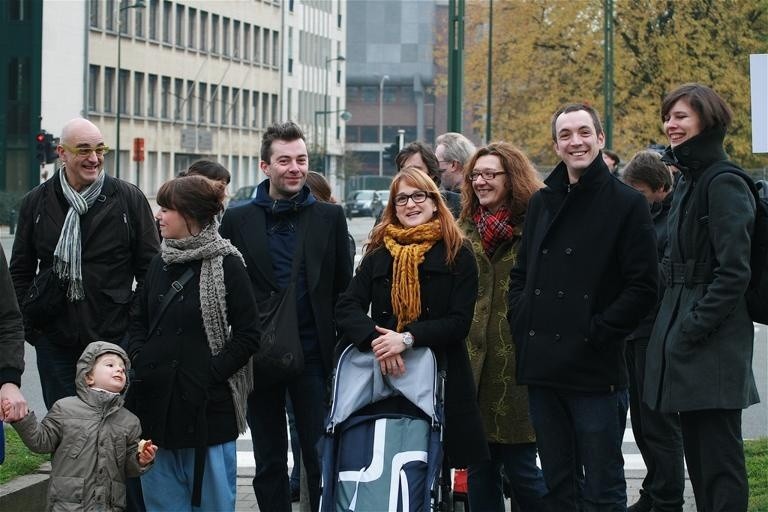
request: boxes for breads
[137,439,152,454]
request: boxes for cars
[375,188,390,208]
[226,186,259,208]
[341,188,382,220]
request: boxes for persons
[1,117,352,511]
[333,82,760,511]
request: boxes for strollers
[308,329,470,512]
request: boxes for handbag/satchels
[249,289,306,383]
[20,257,82,333]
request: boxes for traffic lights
[44,131,61,165]
[35,130,48,165]
[383,134,399,171]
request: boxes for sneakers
[627,488,686,512]
[288,477,301,504]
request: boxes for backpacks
[695,166,768,329]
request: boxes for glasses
[391,192,437,207]
[62,142,110,157]
[469,169,508,182]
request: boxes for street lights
[113,0,148,180]
[376,73,391,176]
[322,52,347,186]
[314,106,352,154]
